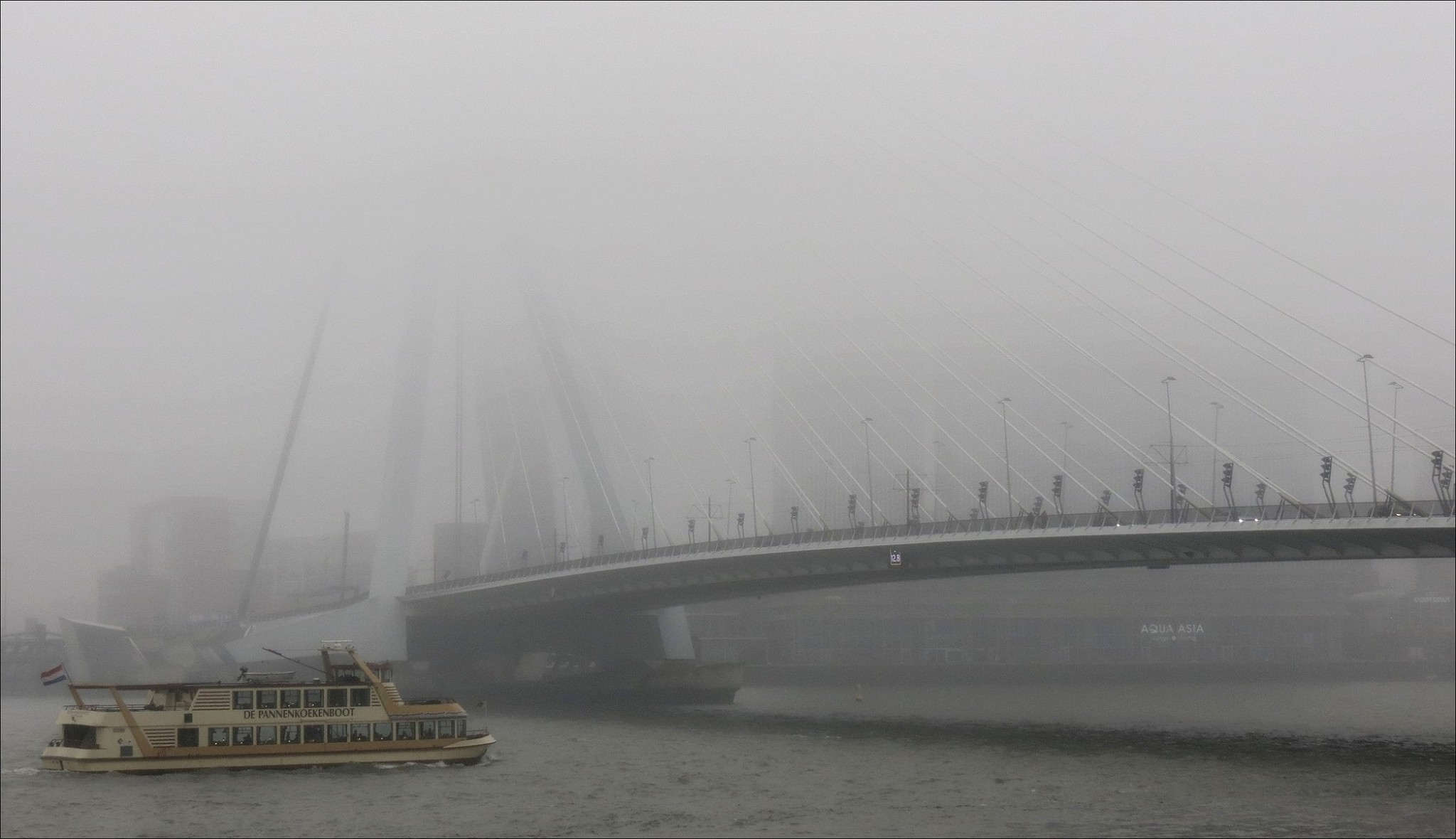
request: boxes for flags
[39,663,70,687]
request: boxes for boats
[236,665,295,684]
[37,640,493,775]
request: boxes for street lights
[861,418,876,527]
[1060,420,1073,514]
[998,396,1014,517]
[726,478,735,539]
[560,476,569,563]
[1161,376,1179,523]
[1389,381,1403,500]
[745,437,758,538]
[644,456,659,547]
[1209,400,1224,507]
[470,498,480,577]
[1358,353,1379,517]
